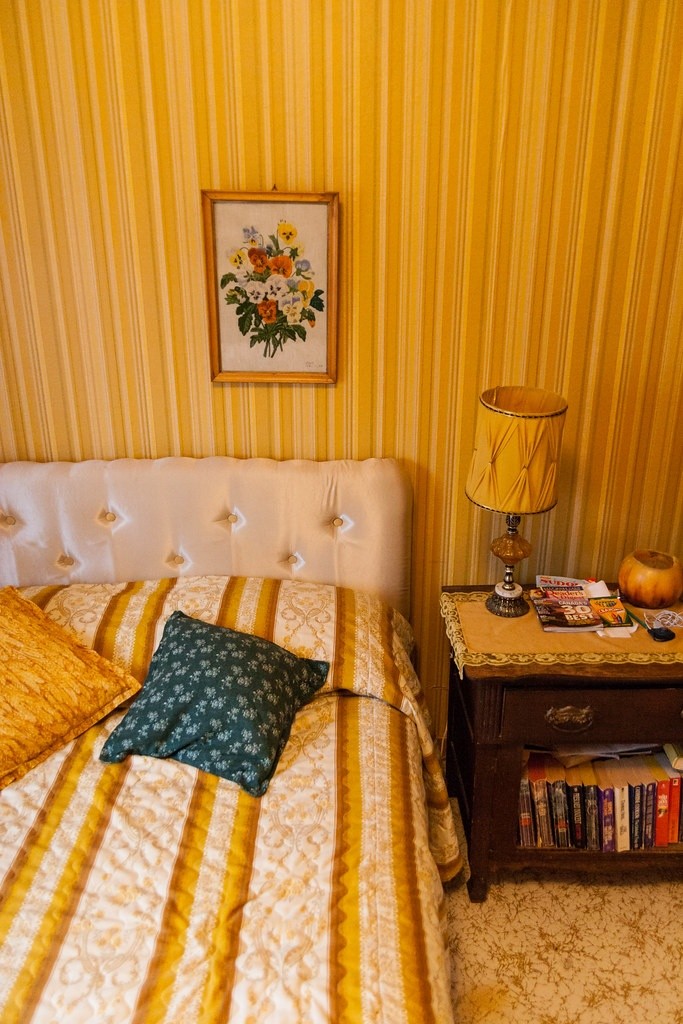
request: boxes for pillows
[0,578,139,794]
[98,610,332,797]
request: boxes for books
[529,575,634,632]
[519,752,680,853]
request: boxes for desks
[439,583,683,903]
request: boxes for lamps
[463,386,569,619]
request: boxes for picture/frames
[200,187,341,385]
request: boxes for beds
[0,455,463,1024]
